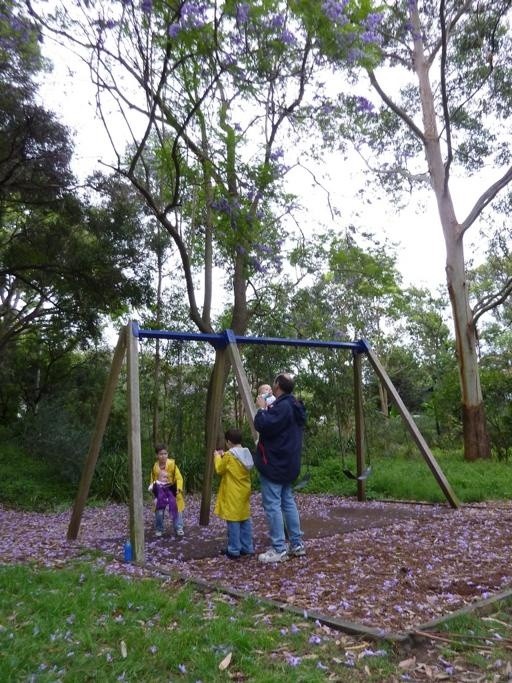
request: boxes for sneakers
[287,542,307,557]
[176,528,184,537]
[257,548,289,564]
[155,531,162,537]
[221,548,240,560]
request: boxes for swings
[140,341,182,498]
[265,344,311,490]
[331,348,373,479]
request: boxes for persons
[251,373,308,565]
[257,384,276,409]
[147,444,185,537]
[212,428,256,559]
[146,468,180,520]
